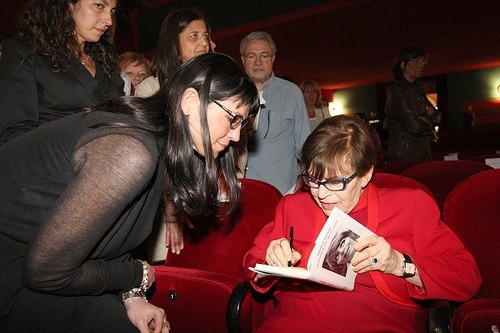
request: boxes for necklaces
[80,55,90,64]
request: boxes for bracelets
[121,287,146,300]
[139,259,150,290]
[124,298,148,307]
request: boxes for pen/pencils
[288,226,293,266]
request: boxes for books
[248,206,379,291]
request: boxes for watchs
[395,252,417,279]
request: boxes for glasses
[208,95,248,130]
[301,169,358,191]
[243,52,273,62]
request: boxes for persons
[382,47,439,161]
[325,230,359,276]
[233,32,310,195]
[0,0,481,333]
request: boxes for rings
[272,263,275,266]
[371,257,377,266]
[163,321,172,330]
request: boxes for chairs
[443,168,500,298]
[380,158,431,174]
[164,177,284,282]
[146,265,252,333]
[453,298,500,333]
[400,158,495,212]
